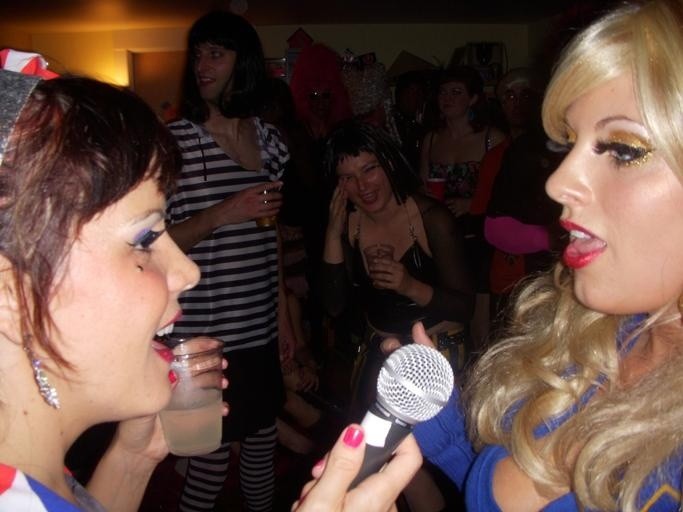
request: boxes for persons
[158,1,682,511]
[0,47,424,512]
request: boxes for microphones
[346,343,455,493]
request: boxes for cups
[256,181,280,230]
[428,177,446,203]
[164,338,226,458]
[362,244,396,266]
